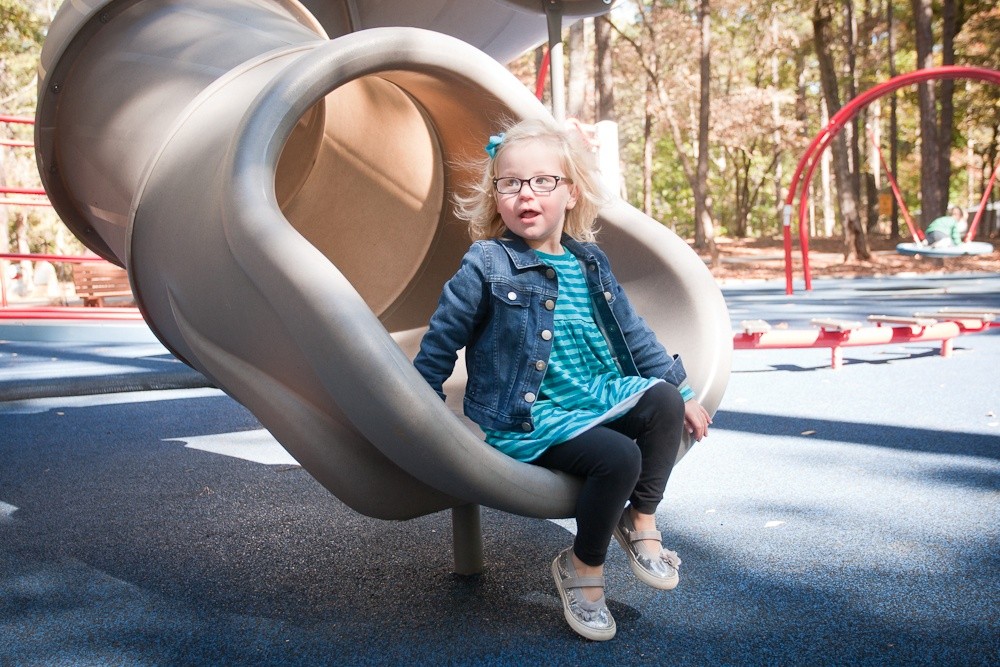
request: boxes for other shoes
[551,545,617,641]
[614,505,681,590]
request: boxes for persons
[924,206,963,270]
[412,119,713,639]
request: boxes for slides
[32,0,733,522]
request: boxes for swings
[856,104,1000,259]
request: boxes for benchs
[72,263,134,308]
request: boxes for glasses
[493,174,573,195]
